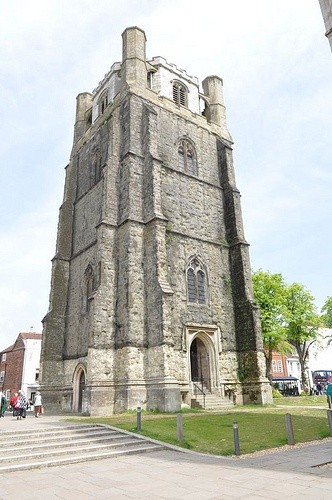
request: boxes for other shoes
[1,413,40,420]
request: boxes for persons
[33,391,42,417]
[0,389,29,420]
[278,371,332,397]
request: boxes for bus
[312,370,332,395]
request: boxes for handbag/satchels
[41,406,44,415]
[23,408,26,417]
